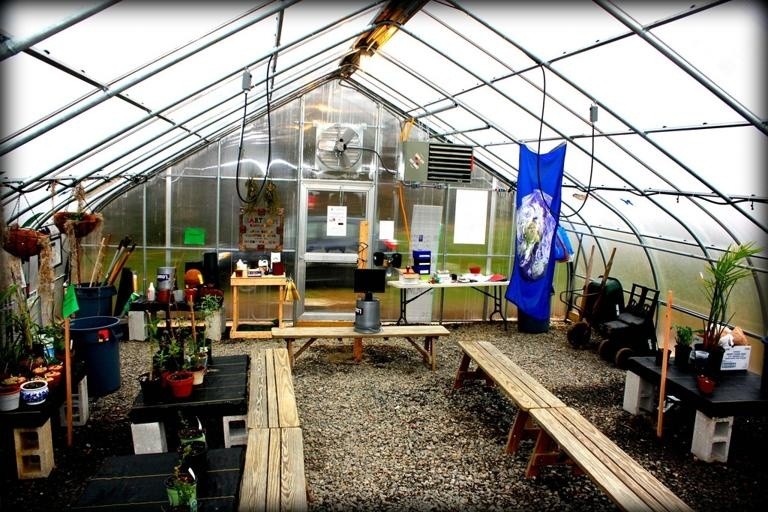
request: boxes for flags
[504,141,567,322]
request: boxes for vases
[20,380,49,405]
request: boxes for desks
[230,272,287,339]
[622,356,768,462]
[386,276,511,334]
[67,445,247,512]
[0,360,95,480]
[127,353,249,453]
[128,297,227,340]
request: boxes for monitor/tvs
[354,269,387,301]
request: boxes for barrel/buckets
[156,266,177,304]
[71,281,119,319]
[258,259,269,272]
[61,315,122,396]
[353,299,382,334]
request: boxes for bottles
[430,272,440,284]
[236,258,243,270]
[146,282,156,301]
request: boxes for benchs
[244,347,301,428]
[454,340,568,453]
[526,408,696,512]
[238,427,307,512]
[271,325,451,371]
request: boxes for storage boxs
[690,329,751,370]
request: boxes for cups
[697,374,715,394]
[241,264,248,278]
[655,347,671,366]
[272,262,283,275]
[696,350,709,367]
[236,270,243,277]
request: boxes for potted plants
[670,323,704,367]
[162,442,200,512]
[175,409,208,449]
[0,184,101,264]
[35,321,75,369]
[137,296,220,396]
[692,238,766,375]
